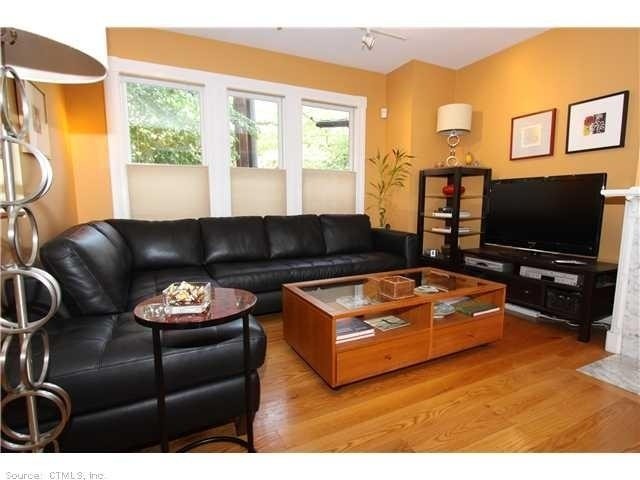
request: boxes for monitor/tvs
[484,173,607,260]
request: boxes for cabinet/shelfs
[417,167,492,270]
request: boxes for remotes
[554,258,593,265]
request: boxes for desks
[459,247,618,342]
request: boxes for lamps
[0,27,108,452]
[436,103,473,167]
[360,27,376,50]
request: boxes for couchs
[0,214,418,453]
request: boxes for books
[335,297,500,342]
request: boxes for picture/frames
[15,80,52,160]
[566,91,628,153]
[510,108,556,160]
